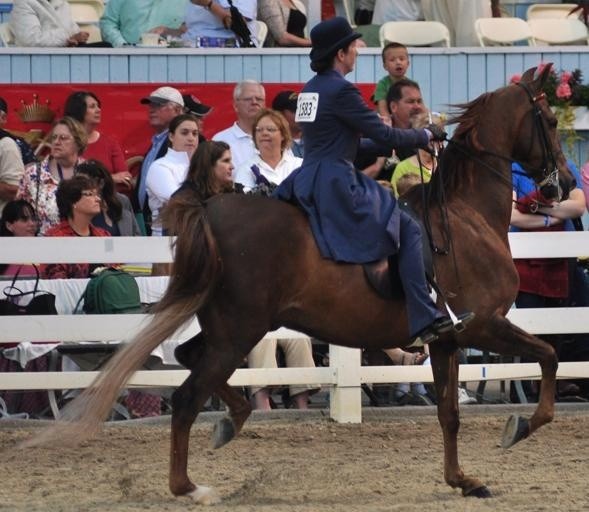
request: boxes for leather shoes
[420,311,476,345]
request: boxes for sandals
[394,351,429,365]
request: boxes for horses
[16,62,563,507]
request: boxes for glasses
[81,191,99,196]
[51,134,73,141]
[255,125,280,132]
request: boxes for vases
[549,106,589,131]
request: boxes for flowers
[509,62,588,106]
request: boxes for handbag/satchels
[0,263,59,316]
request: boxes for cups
[141,34,159,45]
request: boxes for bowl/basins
[199,38,236,49]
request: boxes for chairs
[377,3,589,48]
[0,1,113,49]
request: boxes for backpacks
[83,268,146,314]
[0,129,39,165]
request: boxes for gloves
[424,122,448,143]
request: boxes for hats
[310,17,363,62]
[140,86,185,107]
[183,90,214,117]
[271,89,299,111]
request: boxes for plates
[136,43,170,48]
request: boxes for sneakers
[458,388,477,404]
